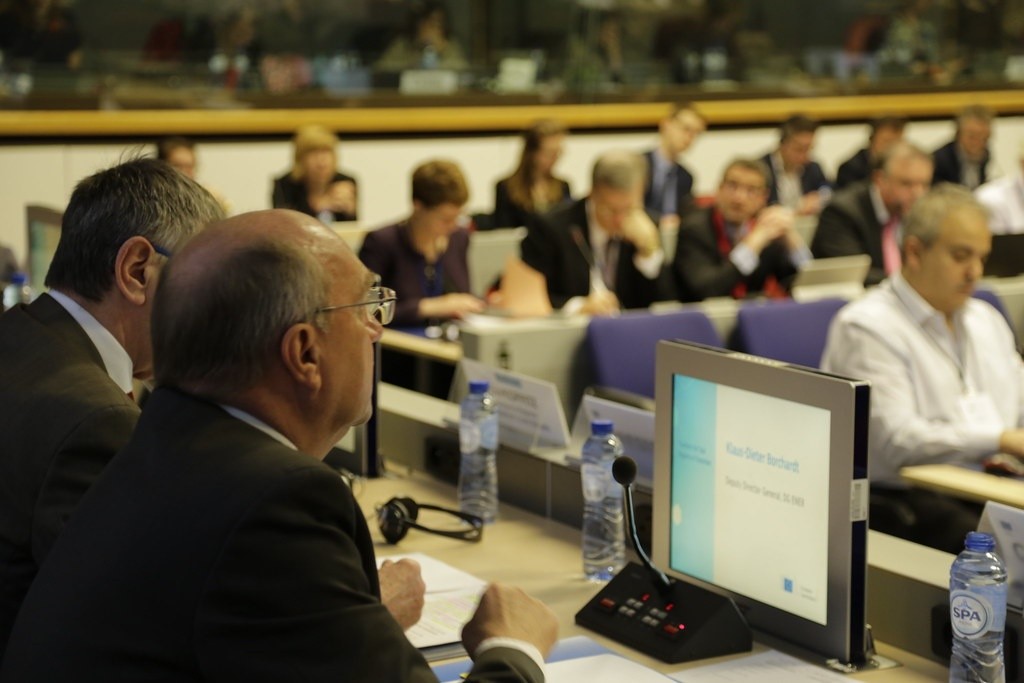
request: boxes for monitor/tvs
[324,274,383,478]
[651,338,871,659]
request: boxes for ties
[882,214,903,277]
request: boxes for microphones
[609,455,670,596]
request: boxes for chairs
[582,310,720,407]
[736,296,917,537]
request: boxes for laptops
[793,255,871,291]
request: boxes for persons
[270,124,358,221]
[156,138,196,175]
[0,157,228,657]
[932,104,993,186]
[836,116,906,188]
[542,148,667,316]
[810,141,935,286]
[673,158,815,303]
[358,160,487,331]
[758,116,828,217]
[641,105,709,229]
[493,122,572,267]
[975,157,1024,236]
[0,208,562,683]
[820,183,1024,556]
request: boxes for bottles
[579,420,625,579]
[948,532,1008,683]
[459,381,498,522]
[3,273,31,310]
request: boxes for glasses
[315,287,397,325]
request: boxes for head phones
[375,497,485,545]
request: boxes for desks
[338,210,1023,683]
